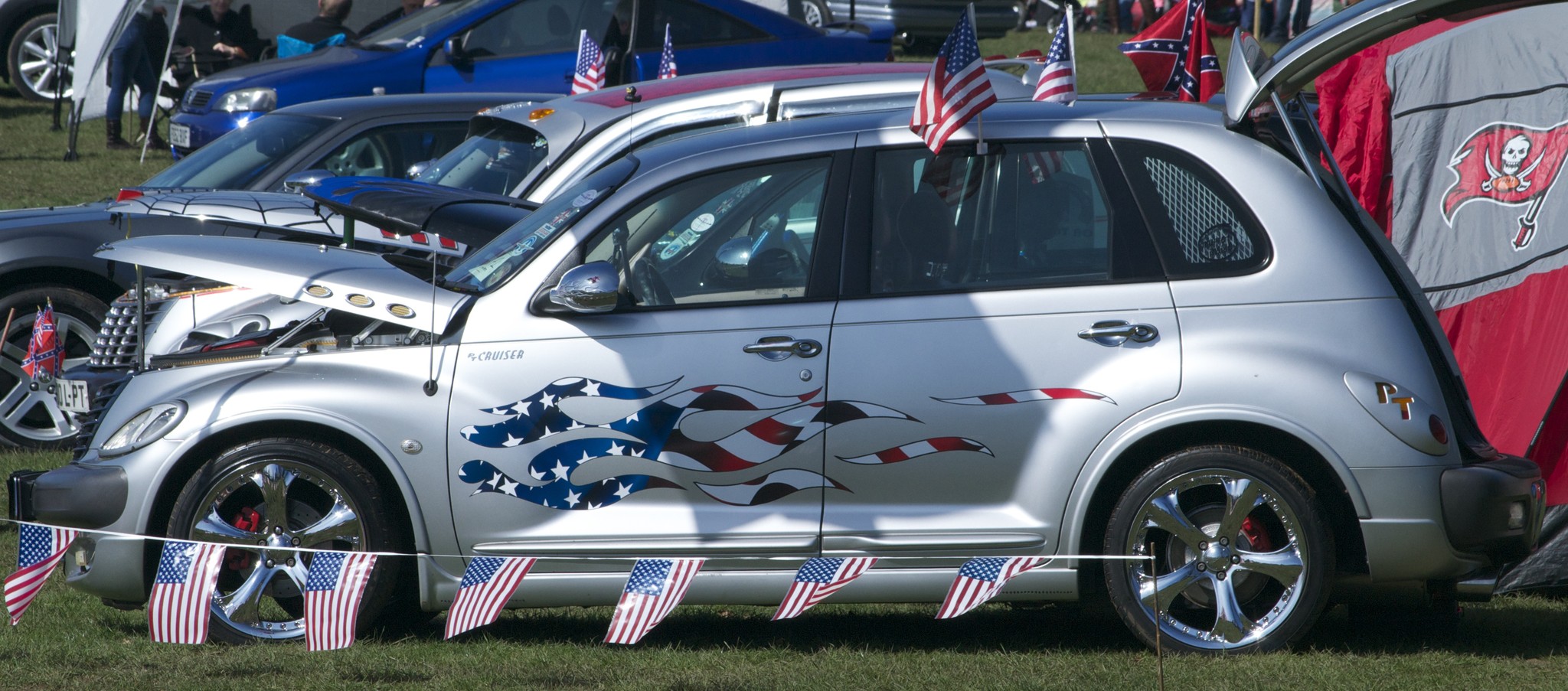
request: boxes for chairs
[123,41,203,145]
[276,31,349,60]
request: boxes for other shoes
[133,135,170,150]
[106,138,141,151]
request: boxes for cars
[0,1,1548,659]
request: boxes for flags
[305,552,377,652]
[446,557,535,639]
[20,307,64,379]
[571,33,604,94]
[602,560,703,644]
[1021,13,1075,186]
[769,558,876,621]
[3,523,80,626]
[150,542,225,645]
[924,140,1005,206]
[935,557,1039,618]
[1117,0,1223,103]
[657,29,678,79]
[909,10,997,153]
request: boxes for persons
[103,0,439,148]
[1046,0,1312,43]
